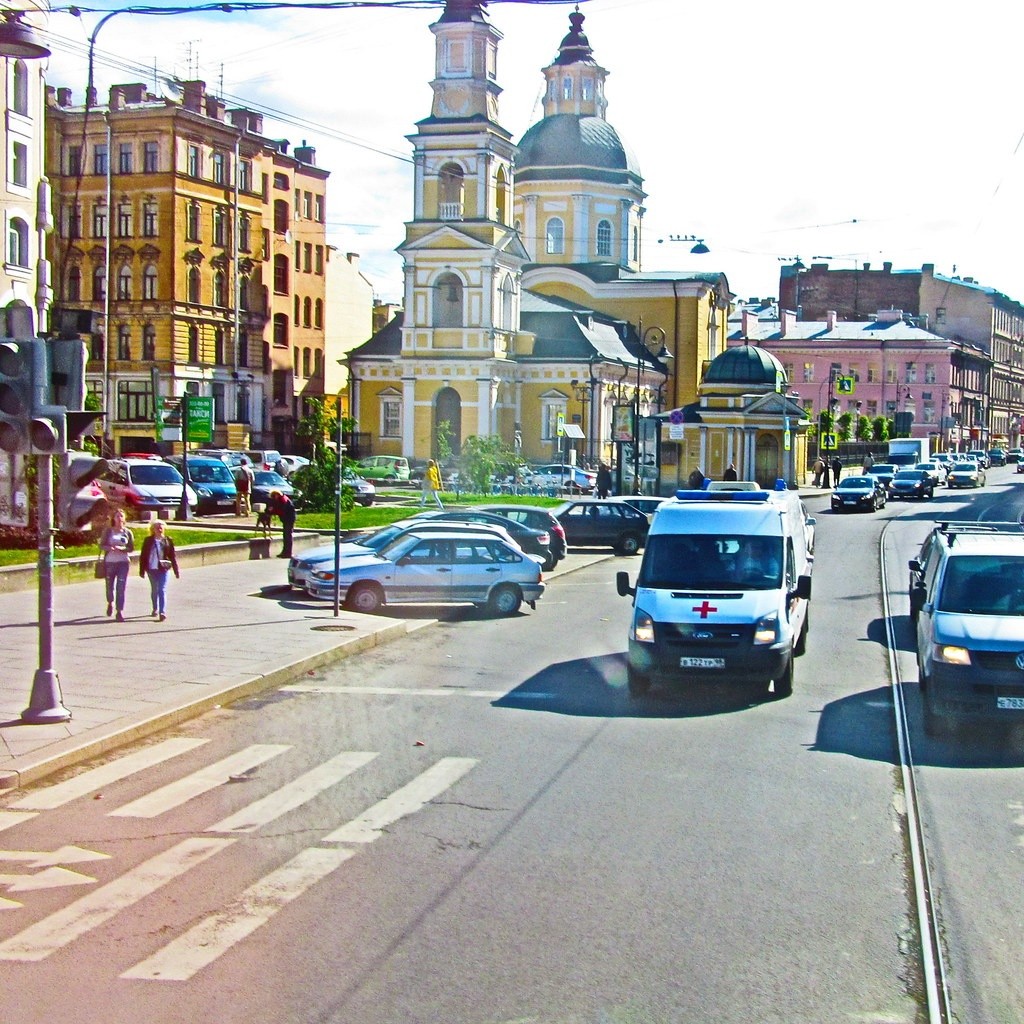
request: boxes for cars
[831,476,887,512]
[917,462,986,488]
[865,463,934,500]
[230,468,304,512]
[930,448,1024,473]
[799,499,816,552]
[281,456,598,507]
[548,497,667,556]
[289,508,553,617]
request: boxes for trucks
[887,437,930,465]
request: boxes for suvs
[909,526,1002,625]
[476,506,568,571]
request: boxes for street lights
[635,316,674,498]
[821,372,914,488]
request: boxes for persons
[268,491,296,558]
[862,452,875,475]
[419,460,444,511]
[812,457,842,488]
[139,519,180,621]
[98,508,135,621]
[725,538,779,580]
[723,463,737,481]
[689,466,704,490]
[595,464,612,499]
[234,459,255,517]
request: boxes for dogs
[255,509,273,539]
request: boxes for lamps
[793,259,805,269]
[0,10,51,59]
[691,239,709,254]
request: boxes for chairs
[436,544,457,561]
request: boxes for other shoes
[116,612,123,621]
[160,613,166,622]
[152,609,158,616]
[107,604,112,616]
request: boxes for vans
[194,448,282,472]
[917,532,1024,737]
[97,458,198,519]
[164,456,239,515]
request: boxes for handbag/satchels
[820,463,824,472]
[236,479,248,491]
[95,561,105,578]
[158,560,171,571]
[593,486,597,498]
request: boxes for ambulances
[617,477,814,696]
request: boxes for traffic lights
[0,337,68,456]
